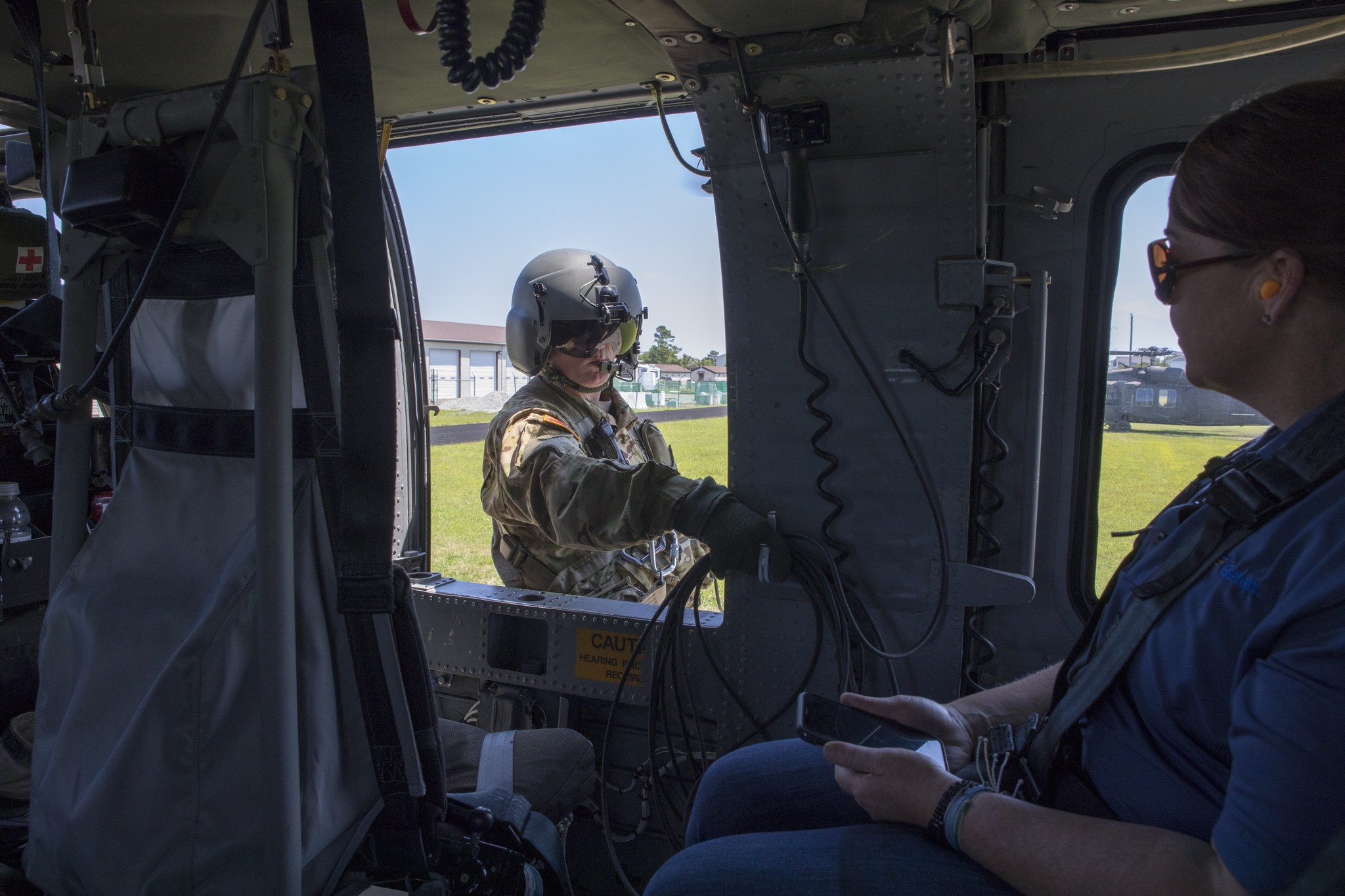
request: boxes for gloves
[699,495,789,583]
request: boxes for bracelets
[928,779,980,844]
[943,785,993,850]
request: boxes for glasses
[1148,237,1267,306]
[549,314,639,358]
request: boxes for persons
[646,79,1345,896]
[479,248,789,601]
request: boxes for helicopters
[1104,346,1272,432]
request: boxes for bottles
[0,481,32,544]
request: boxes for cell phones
[794,691,952,776]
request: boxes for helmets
[505,249,643,377]
